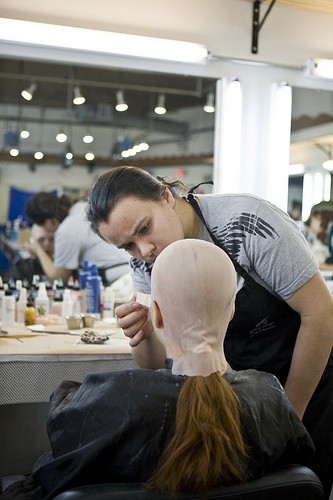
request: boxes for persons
[0,239,320,500]
[0,190,130,280]
[85,166,333,500]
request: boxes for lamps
[20,83,37,101]
[154,93,166,116]
[203,92,215,114]
[115,90,128,113]
[72,86,86,106]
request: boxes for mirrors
[286,86,333,286]
[0,55,217,316]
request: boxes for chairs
[54,465,324,500]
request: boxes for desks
[0,315,139,405]
